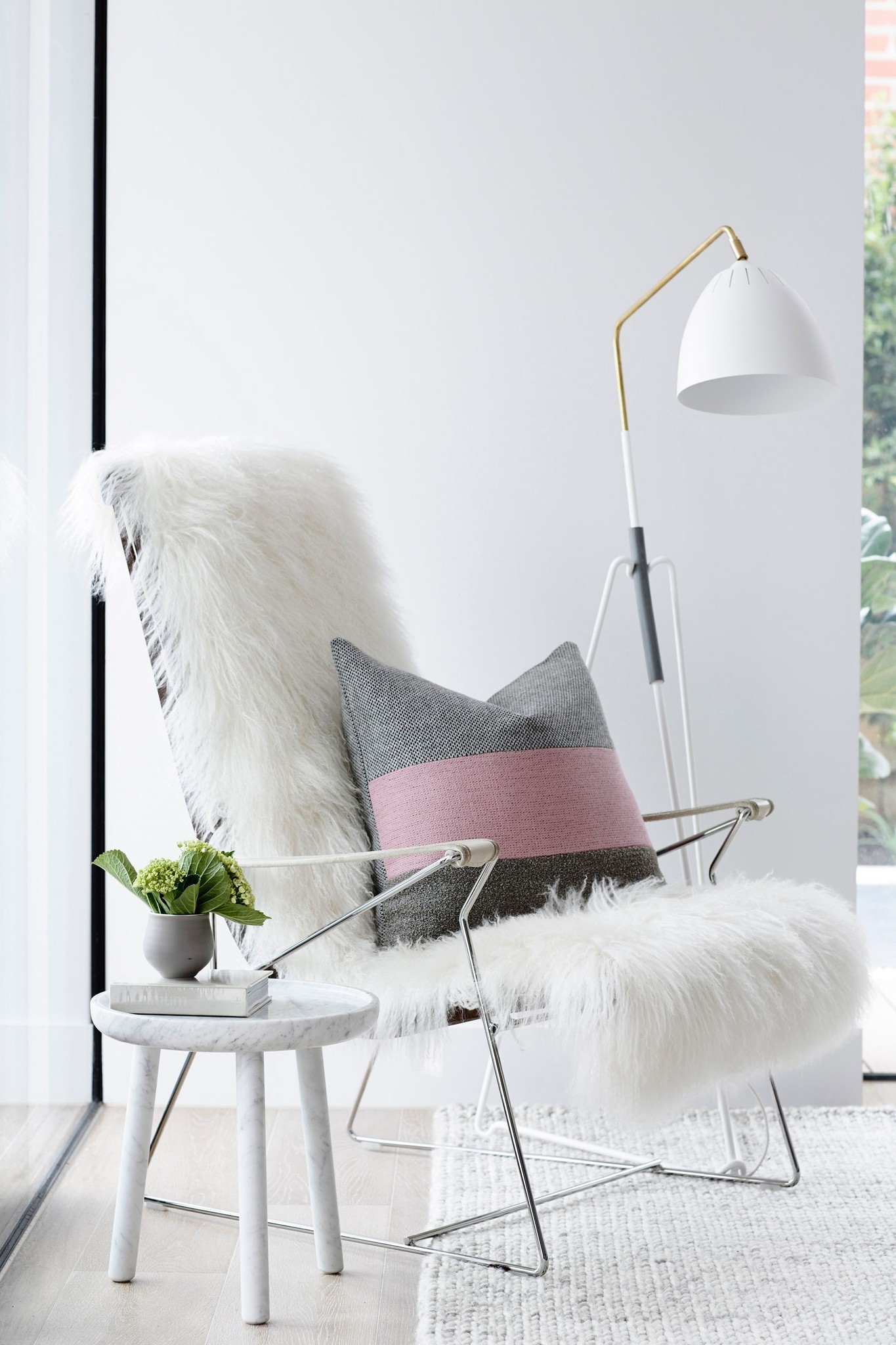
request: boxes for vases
[144,912,215,979]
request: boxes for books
[109,966,274,1017]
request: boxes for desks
[91,981,380,1326]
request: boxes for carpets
[413,1106,896,1345]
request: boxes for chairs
[69,434,870,1278]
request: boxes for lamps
[474,222,837,1185]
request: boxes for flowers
[93,840,273,926]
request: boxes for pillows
[331,638,665,952]
